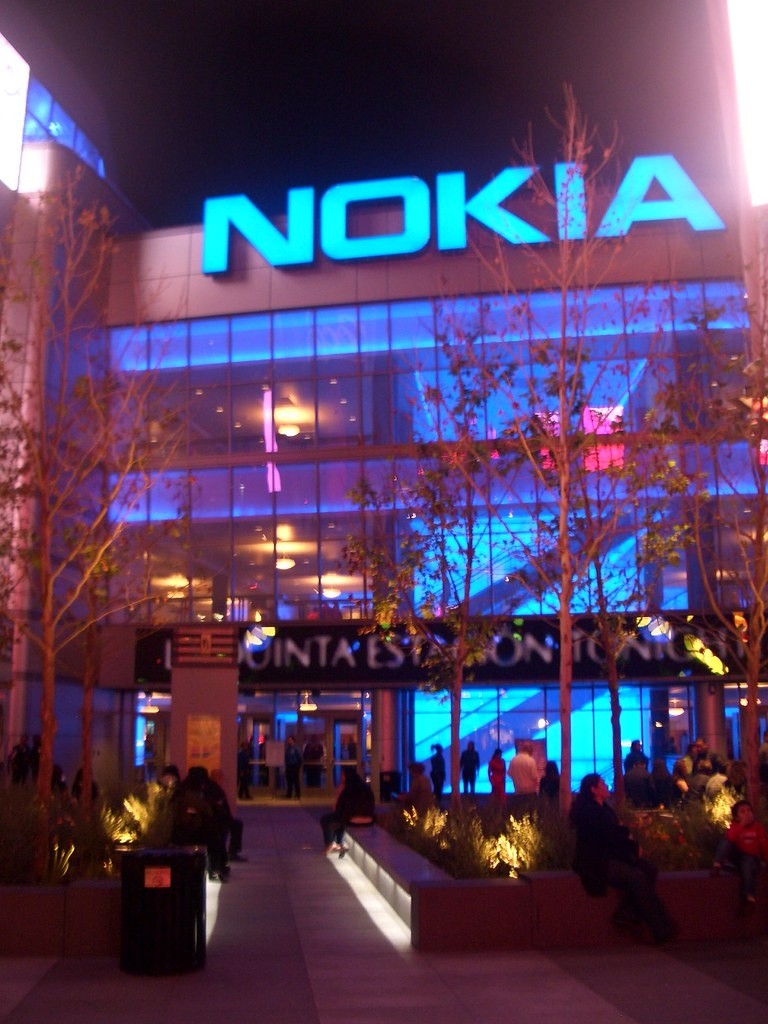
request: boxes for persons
[52,764,64,796]
[9,735,30,788]
[507,743,561,800]
[460,741,480,795]
[713,800,768,902]
[154,767,248,882]
[624,740,648,775]
[570,774,679,947]
[430,745,448,811]
[286,736,302,799]
[489,749,508,798]
[671,729,768,805]
[624,758,682,808]
[32,734,41,783]
[398,762,433,817]
[319,768,375,857]
[304,733,324,788]
[238,742,252,801]
[71,768,97,805]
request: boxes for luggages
[118,845,207,975]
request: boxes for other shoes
[229,853,246,860]
[737,898,756,915]
[330,843,349,860]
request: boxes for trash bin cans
[121,845,206,978]
